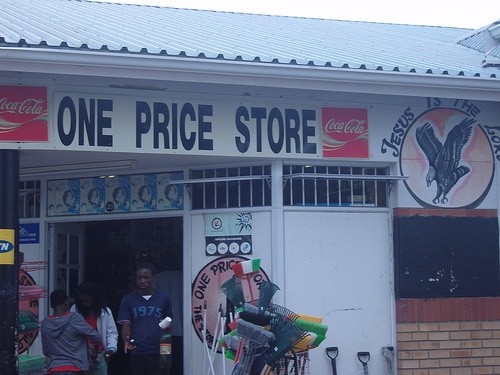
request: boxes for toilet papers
[158,317,172,329]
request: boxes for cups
[159,317,172,328]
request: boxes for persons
[40,289,102,375]
[69,260,183,375]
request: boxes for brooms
[201,258,328,375]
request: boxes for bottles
[160,332,171,368]
